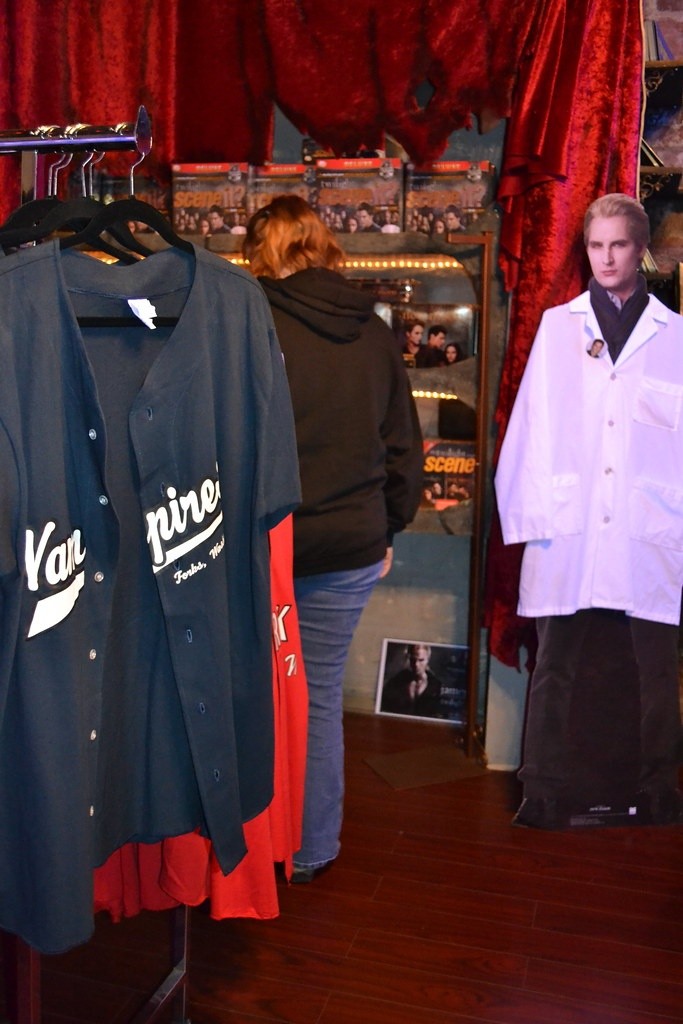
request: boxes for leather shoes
[282,858,335,883]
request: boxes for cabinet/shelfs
[0,126,135,262]
[50,123,196,264]
[1,124,154,259]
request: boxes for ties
[414,674,423,699]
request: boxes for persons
[381,643,443,718]
[586,338,604,359]
[443,342,463,365]
[241,194,426,883]
[497,192,682,828]
[414,324,448,368]
[399,318,426,368]
[127,201,480,237]
